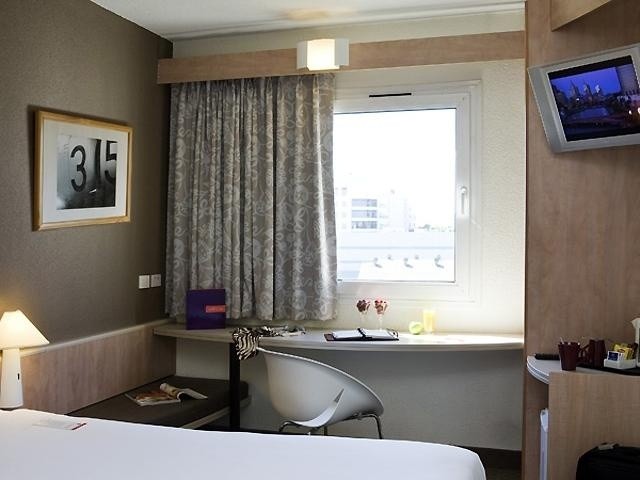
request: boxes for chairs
[254,347,384,440]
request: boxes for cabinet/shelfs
[64,375,251,430]
[526,356,640,480]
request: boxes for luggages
[575,440,638,479]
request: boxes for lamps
[0,308,51,410]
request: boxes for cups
[558,341,580,371]
[584,339,604,368]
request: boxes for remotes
[533,353,560,360]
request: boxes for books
[125,382,208,407]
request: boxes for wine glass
[374,297,388,329]
[354,296,372,329]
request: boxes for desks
[153,323,524,432]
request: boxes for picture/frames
[30,106,135,232]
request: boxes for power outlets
[152,274,163,288]
[138,274,151,289]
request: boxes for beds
[1,408,488,480]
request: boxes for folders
[185,288,227,332]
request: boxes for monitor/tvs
[526,46,636,153]
